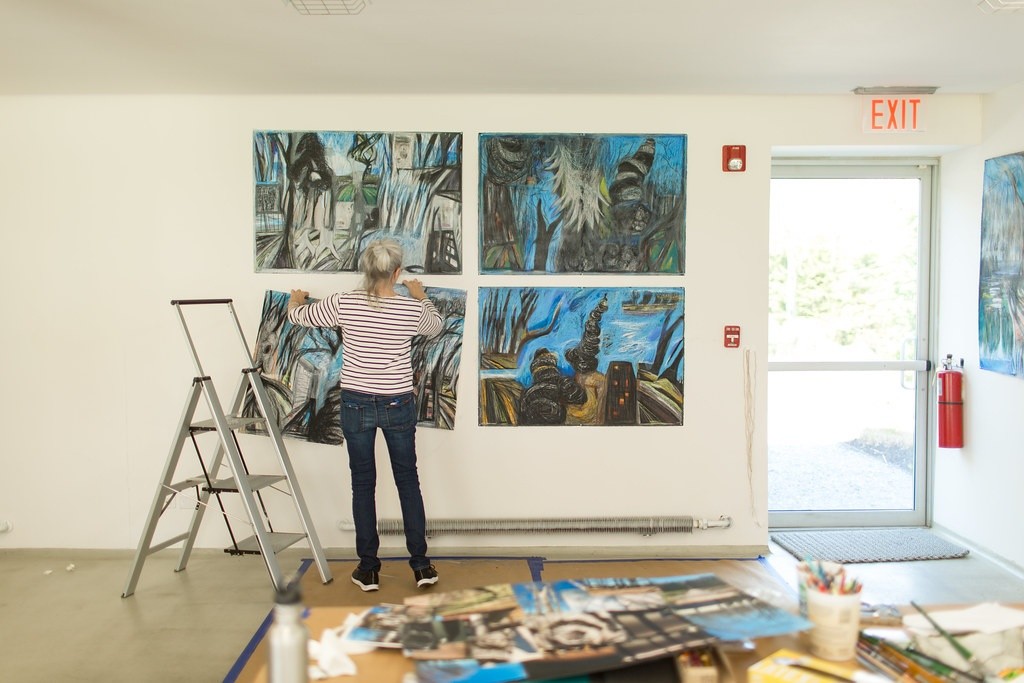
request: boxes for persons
[287,239,443,593]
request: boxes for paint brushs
[910,599,1003,683]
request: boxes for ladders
[120,299,333,597]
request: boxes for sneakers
[351,565,379,591]
[414,564,438,587]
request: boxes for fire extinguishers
[931,353,966,448]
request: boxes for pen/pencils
[798,554,865,596]
[855,627,965,683]
